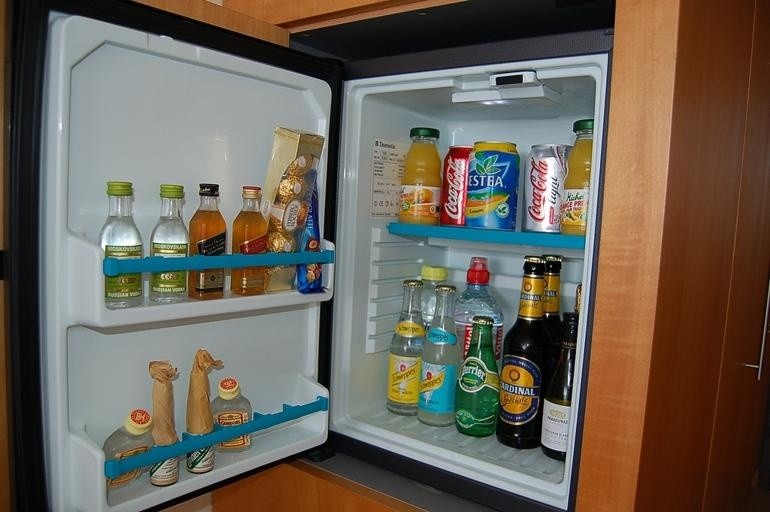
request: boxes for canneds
[441,146,472,226]
[466,141,520,230]
[524,144,573,233]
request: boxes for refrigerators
[0,0,618,512]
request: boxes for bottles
[148,184,190,306]
[453,316,500,436]
[210,377,252,453]
[231,186,267,294]
[190,183,226,299]
[103,408,155,491]
[149,360,179,486]
[399,127,443,226]
[540,312,578,460]
[417,285,464,428]
[559,118,594,237]
[496,256,548,449]
[385,279,426,415]
[419,264,448,330]
[100,181,144,309]
[541,253,565,387]
[455,257,504,377]
[185,348,224,474]
